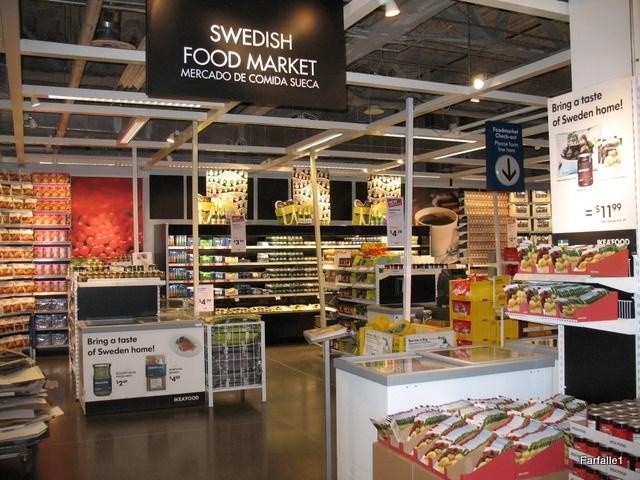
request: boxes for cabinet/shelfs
[0,172,32,366]
[67,264,168,402]
[464,191,550,278]
[152,223,431,341]
[204,319,267,408]
[33,170,72,362]
[495,239,640,480]
[329,252,465,355]
[448,278,518,345]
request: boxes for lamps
[166,132,175,144]
[469,96,481,104]
[30,119,39,129]
[382,0,401,17]
[472,76,484,90]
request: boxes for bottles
[146,355,167,392]
[628,421,640,443]
[79,273,87,282]
[135,272,143,278]
[613,419,629,440]
[149,264,156,271]
[143,272,149,278]
[154,270,159,277]
[73,260,103,272]
[587,411,602,431]
[148,271,154,277]
[110,273,117,279]
[602,410,640,421]
[587,400,640,410]
[93,273,99,279]
[598,415,614,435]
[105,272,110,279]
[88,273,92,279]
[130,272,135,278]
[160,271,165,279]
[123,272,130,278]
[577,153,594,188]
[112,255,131,263]
[137,265,144,271]
[117,272,123,279]
[99,272,105,279]
[130,265,136,272]
[124,265,130,272]
